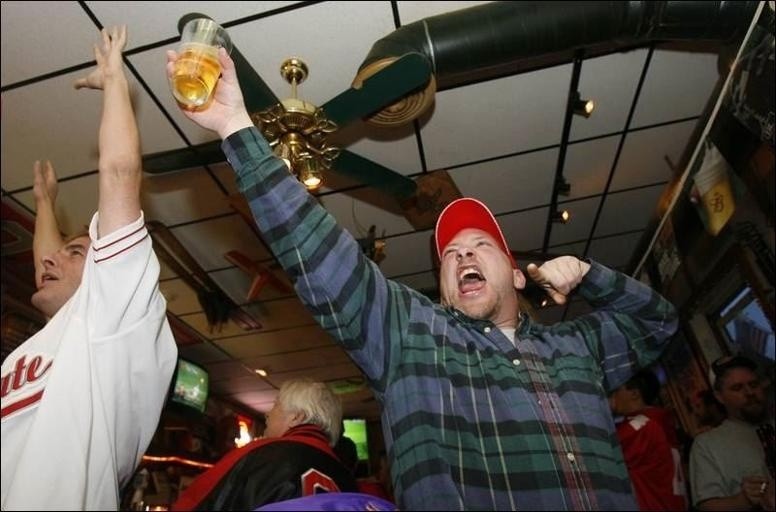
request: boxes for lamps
[264,131,327,197]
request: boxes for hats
[435,198,516,268]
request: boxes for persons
[693,390,723,427]
[166,48,680,512]
[688,354,776,512]
[171,377,362,512]
[613,368,690,512]
[1,25,180,511]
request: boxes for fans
[139,12,430,206]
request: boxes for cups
[169,18,234,108]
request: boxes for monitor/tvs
[341,417,374,470]
[171,358,210,416]
